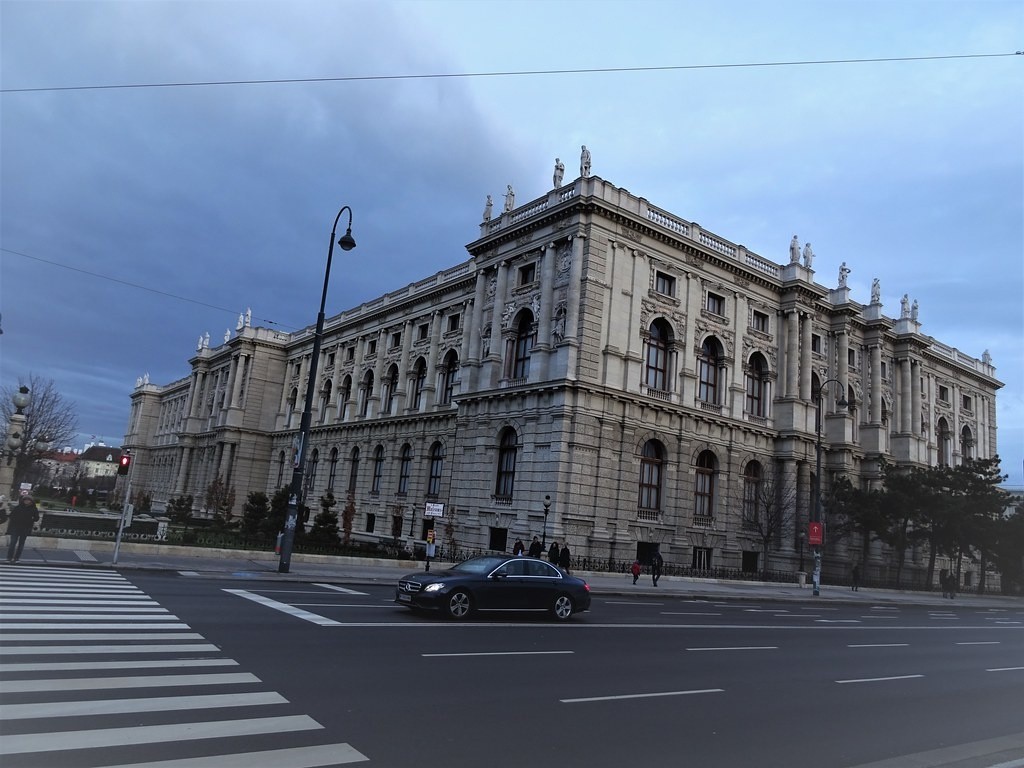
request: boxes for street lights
[408,502,417,535]
[798,528,805,572]
[814,379,849,595]
[278,206,356,574]
[541,495,552,552]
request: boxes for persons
[553,158,565,189]
[651,552,663,587]
[790,236,815,268]
[900,294,910,317]
[6,495,39,561]
[852,566,860,591]
[483,195,493,221]
[911,299,918,319]
[548,542,559,566]
[529,536,542,558]
[838,262,850,288]
[871,278,880,302]
[559,542,570,575]
[581,146,591,178]
[196,307,251,350]
[502,185,515,212]
[137,372,149,386]
[513,537,524,555]
[631,560,640,585]
[982,350,991,364]
[942,573,956,599]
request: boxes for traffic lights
[117,455,131,476]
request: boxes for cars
[395,554,592,623]
